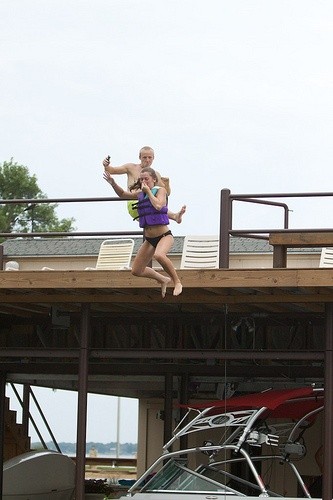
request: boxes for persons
[104,166,182,298]
[104,146,186,224]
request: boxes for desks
[269,232,333,269]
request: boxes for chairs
[180,233,219,270]
[319,248,333,268]
[85,238,135,270]
